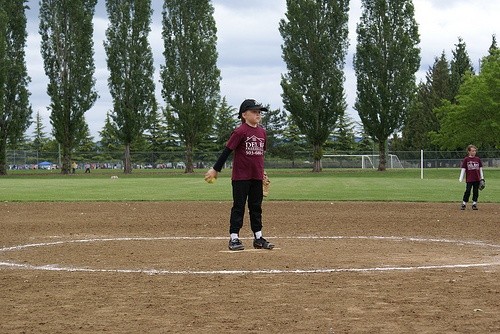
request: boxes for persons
[72,161,77,174]
[459,144,485,210]
[205,99,274,250]
[85,162,91,173]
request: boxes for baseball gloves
[478,179,485,190]
[263,171,270,197]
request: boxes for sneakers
[253,237,275,249]
[228,238,244,251]
[472,204,478,210]
[460,205,466,210]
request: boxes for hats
[238,99,268,113]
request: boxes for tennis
[209,177,216,183]
